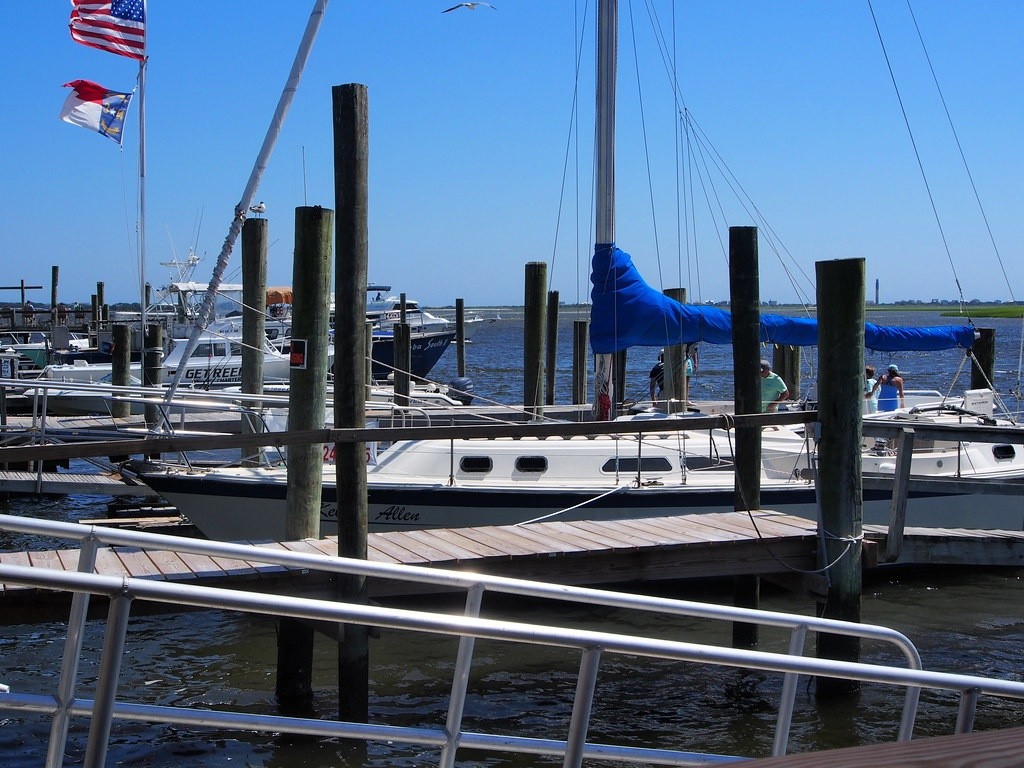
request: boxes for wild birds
[440,2,498,13]
[250,201,266,218]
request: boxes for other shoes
[686,401,697,406]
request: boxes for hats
[760,359,771,373]
[658,348,665,360]
[888,364,899,373]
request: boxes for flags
[68,0,146,61]
[61,80,132,145]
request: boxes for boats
[0,204,465,416]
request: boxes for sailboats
[118,0,1024,568]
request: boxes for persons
[864,364,905,413]
[761,360,789,413]
[649,349,664,407]
[685,342,698,405]
